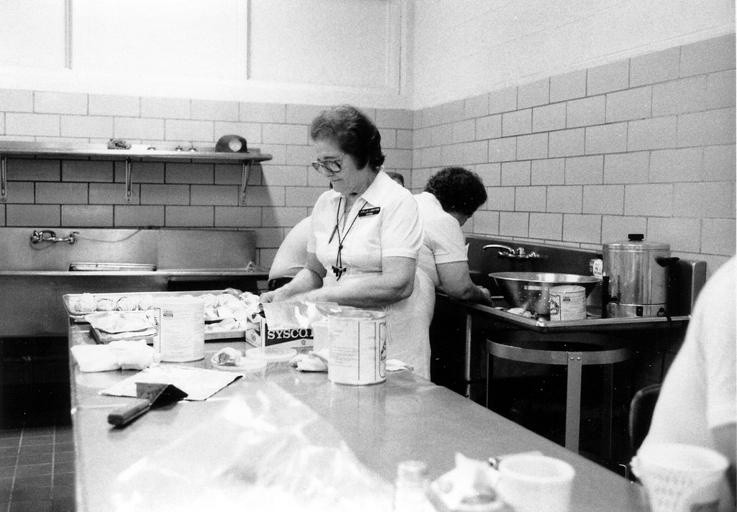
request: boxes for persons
[412,167,496,382]
[259,104,432,382]
[629,255,736,512]
[386,171,405,188]
[267,181,333,291]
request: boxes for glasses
[311,153,346,178]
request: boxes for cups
[498,455,576,512]
[637,442,730,512]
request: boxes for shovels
[108,382,188,426]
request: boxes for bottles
[393,461,433,512]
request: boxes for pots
[603,233,681,316]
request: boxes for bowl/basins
[487,272,600,316]
